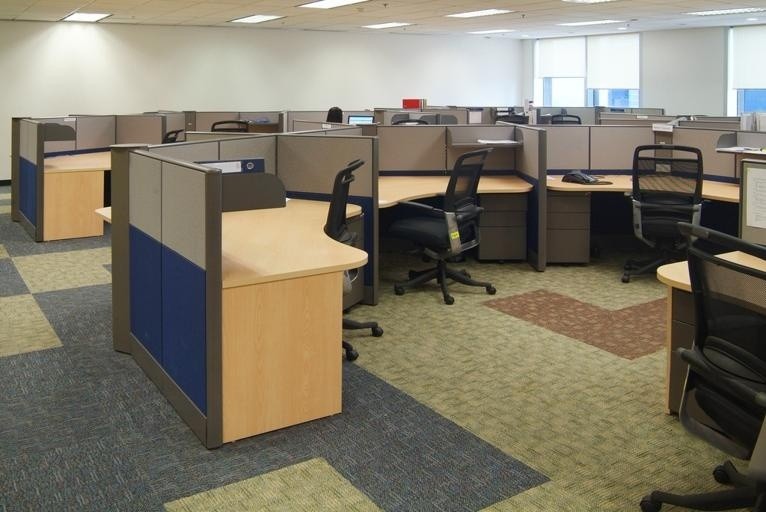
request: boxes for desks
[546,174,740,264]
[43,150,111,241]
[221,195,368,443]
[378,175,534,261]
[95,207,111,223]
[656,247,766,435]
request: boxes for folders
[193,159,264,175]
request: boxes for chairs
[325,159,383,362]
[623,145,711,282]
[495,114,581,125]
[675,222,766,461]
[392,120,428,125]
[211,120,248,133]
[162,128,185,143]
[395,149,495,304]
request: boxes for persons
[326,107,342,123]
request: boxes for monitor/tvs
[347,116,375,125]
